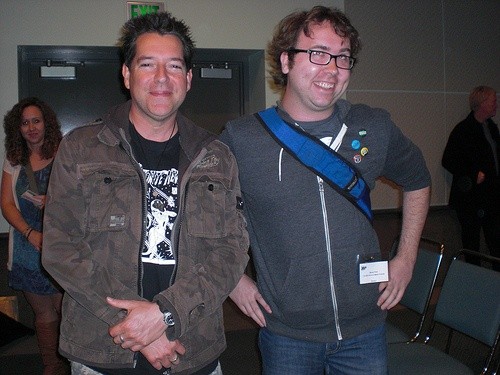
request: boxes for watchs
[156,300,175,326]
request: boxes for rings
[119,335,124,343]
[169,354,178,363]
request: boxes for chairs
[388,249,500,374]
[386,234,446,343]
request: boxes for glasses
[286,45,357,70]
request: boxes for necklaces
[127,118,177,185]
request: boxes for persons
[216,5,431,375]
[42,10,250,375]
[440,85,500,267]
[0,96,63,375]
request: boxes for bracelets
[23,225,34,241]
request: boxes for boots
[34,317,64,374]
